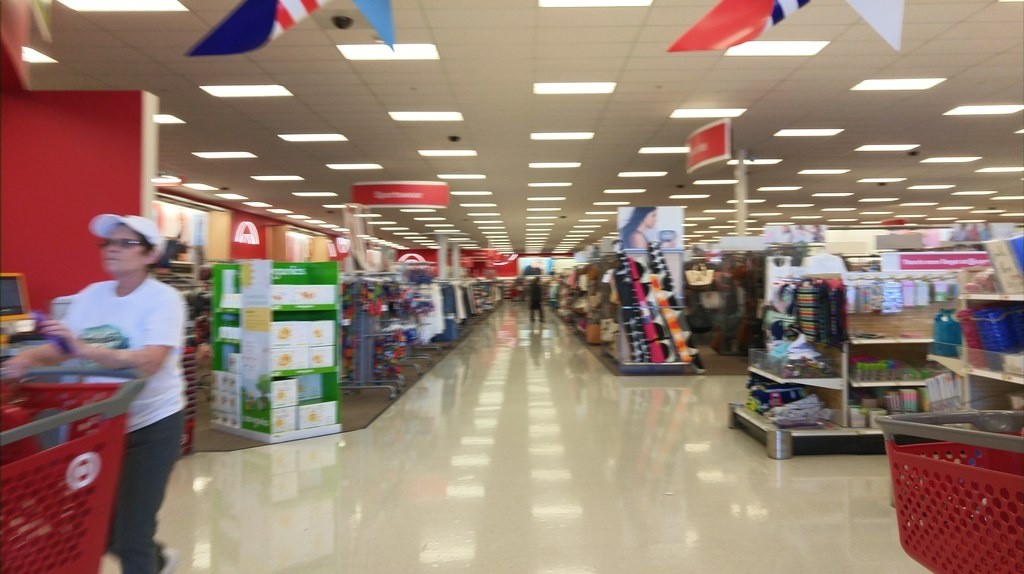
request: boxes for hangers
[382,318,419,332]
[399,278,475,290]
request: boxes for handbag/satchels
[686,305,713,334]
[699,286,723,309]
[686,262,714,285]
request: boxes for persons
[622,207,676,248]
[952,224,990,240]
[0,214,187,574]
[523,261,541,275]
[780,226,824,242]
[530,277,545,322]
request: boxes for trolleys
[0,332,146,574]
[875,410,1024,574]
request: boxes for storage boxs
[211,285,338,433]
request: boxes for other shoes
[158,550,178,574]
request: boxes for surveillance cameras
[907,152,920,157]
[677,185,685,189]
[447,136,461,142]
[219,186,229,191]
[332,16,352,29]
[878,182,888,186]
[559,216,566,218]
[328,211,334,213]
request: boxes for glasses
[96,238,150,249]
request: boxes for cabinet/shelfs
[503,205,1024,574]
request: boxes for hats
[88,214,164,246]
